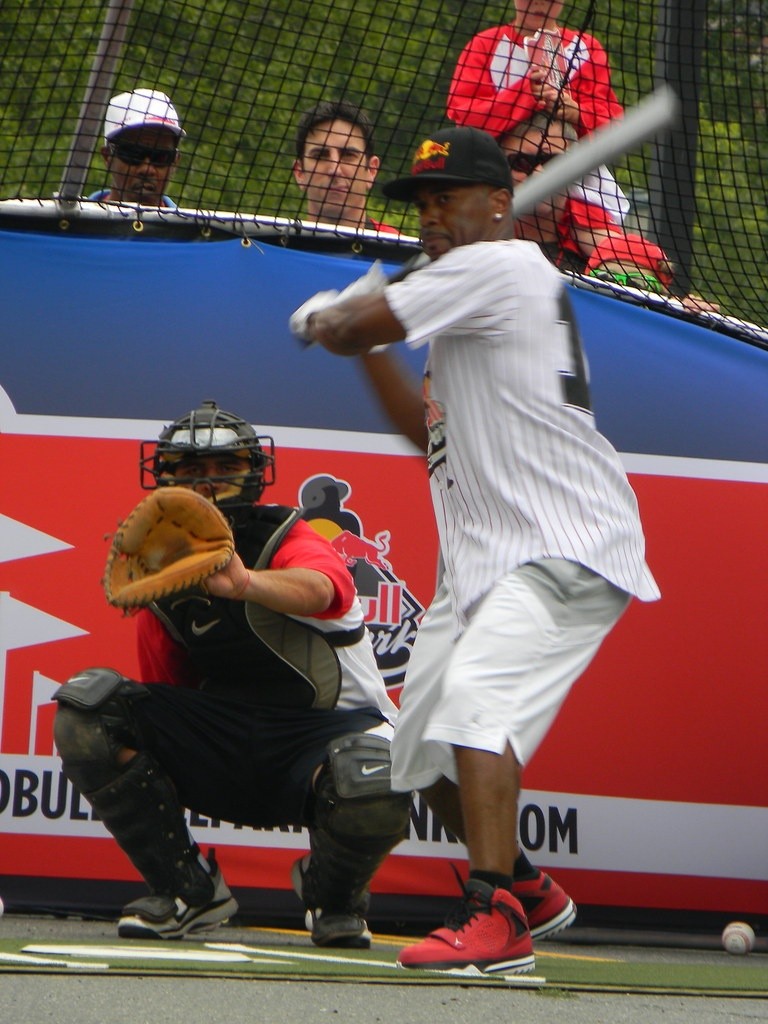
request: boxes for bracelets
[233,570,251,601]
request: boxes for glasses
[107,142,176,168]
[506,152,558,173]
[590,270,661,293]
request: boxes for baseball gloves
[102,483,235,611]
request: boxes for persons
[87,88,186,208]
[51,399,415,945]
[293,101,403,235]
[445,0,720,313]
[290,127,662,973]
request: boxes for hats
[103,88,186,146]
[381,126,514,201]
[586,234,675,290]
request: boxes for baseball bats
[288,83,678,340]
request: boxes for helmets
[138,399,276,534]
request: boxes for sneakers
[396,878,535,975]
[291,854,372,948]
[118,847,238,939]
[467,868,577,941]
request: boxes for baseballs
[721,920,756,955]
